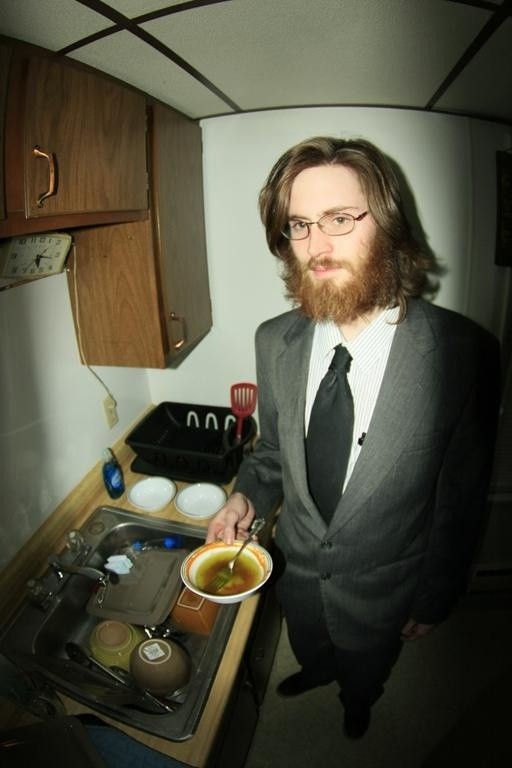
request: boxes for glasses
[274,211,370,242]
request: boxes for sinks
[0,506,241,742]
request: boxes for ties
[306,344,354,524]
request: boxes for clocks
[0,233,72,279]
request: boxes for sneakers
[276,667,334,696]
[344,704,371,739]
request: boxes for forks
[204,517,267,594]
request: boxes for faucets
[49,559,110,589]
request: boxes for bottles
[101,448,125,500]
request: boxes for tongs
[57,638,178,716]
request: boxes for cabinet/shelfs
[206,591,283,768]
[66,100,214,370]
[1,37,149,239]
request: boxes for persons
[201,128,505,740]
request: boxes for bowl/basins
[173,483,229,520]
[128,637,191,697]
[171,586,221,636]
[88,620,148,675]
[180,540,274,606]
[127,476,177,514]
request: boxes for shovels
[231,382,257,446]
